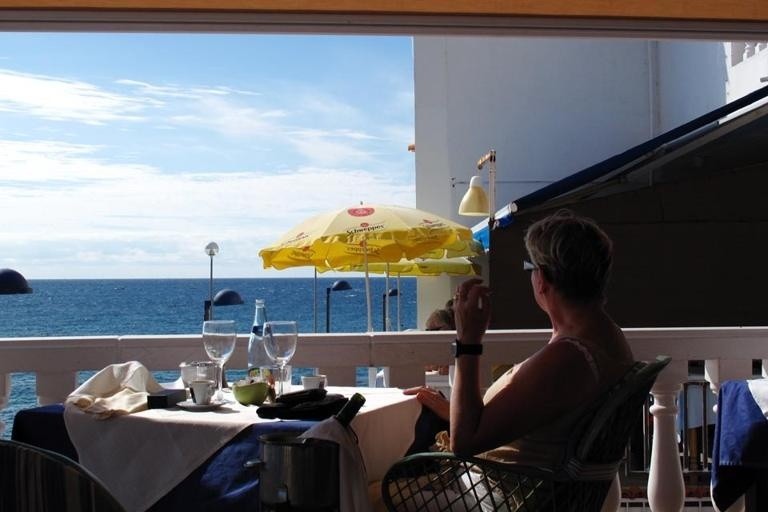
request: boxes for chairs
[381,354,671,511]
[0,439,125,512]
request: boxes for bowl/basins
[232,382,268,406]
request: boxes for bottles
[247,299,275,384]
[302,392,366,442]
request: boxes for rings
[453,292,466,300]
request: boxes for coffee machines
[180,361,219,404]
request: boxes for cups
[301,373,329,392]
[274,365,292,395]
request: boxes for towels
[66,359,162,420]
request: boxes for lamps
[459,169,488,216]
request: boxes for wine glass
[262,320,298,401]
[202,320,236,403]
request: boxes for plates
[176,401,225,411]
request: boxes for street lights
[205,241,219,318]
[382,288,404,331]
[459,149,497,327]
[324,280,351,334]
[204,290,244,334]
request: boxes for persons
[402,213,636,512]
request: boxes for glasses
[524,261,548,271]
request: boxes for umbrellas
[257,201,484,331]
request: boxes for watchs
[450,338,484,358]
